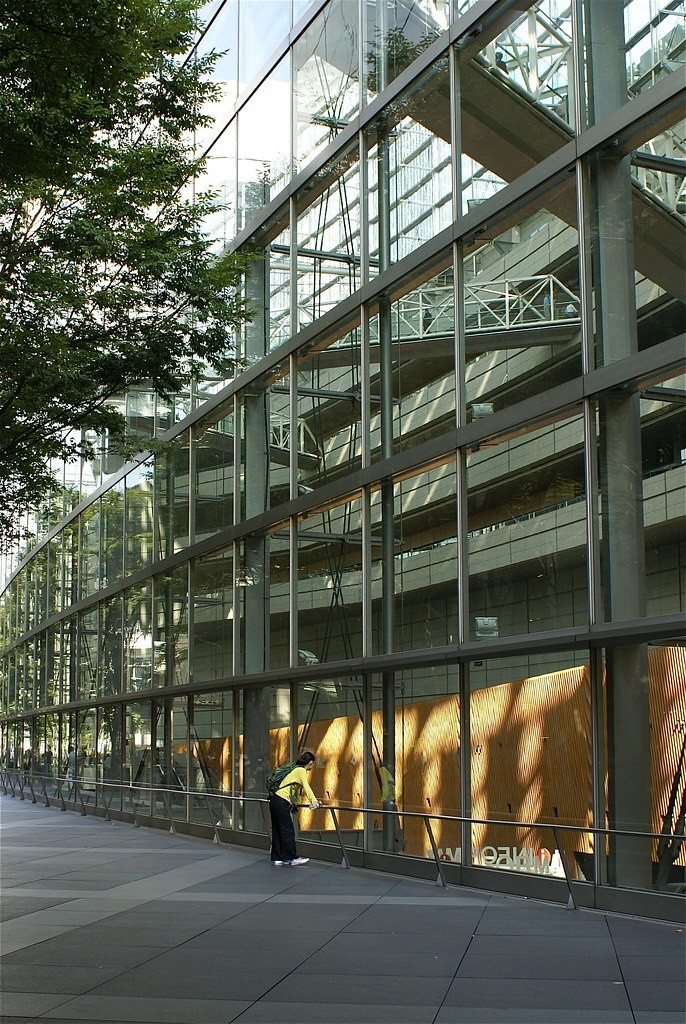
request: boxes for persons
[379,760,405,850]
[2,744,101,788]
[265,752,321,866]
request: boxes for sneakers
[290,857,309,865]
[274,860,290,865]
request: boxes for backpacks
[265,761,305,800]
